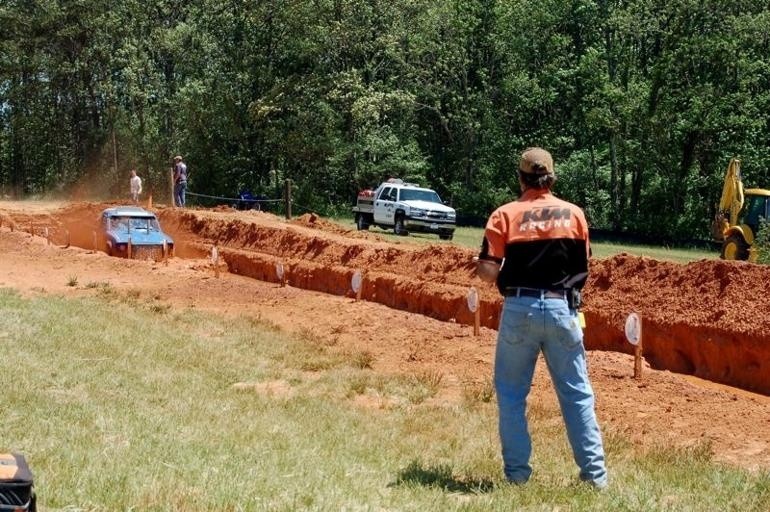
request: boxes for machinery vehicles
[710,156,770,264]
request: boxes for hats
[173,156,183,161]
[519,147,555,176]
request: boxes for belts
[504,288,565,300]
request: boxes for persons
[129,167,144,204]
[171,155,189,207]
[467,144,610,491]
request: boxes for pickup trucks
[86,205,175,261]
[350,181,457,240]
